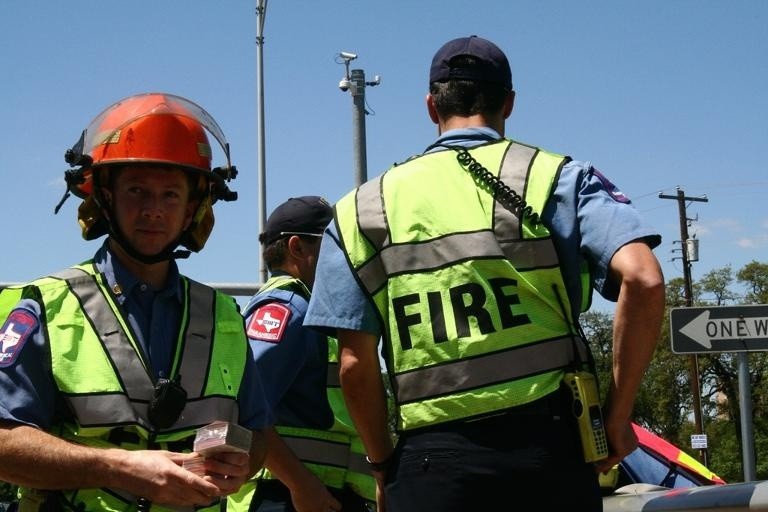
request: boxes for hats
[262,196,334,246]
[430,35,513,91]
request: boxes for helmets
[65,93,238,201]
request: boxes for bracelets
[364,453,397,471]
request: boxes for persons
[240,195,379,512]
[0,95,269,512]
[303,37,667,511]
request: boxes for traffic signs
[668,302,767,356]
